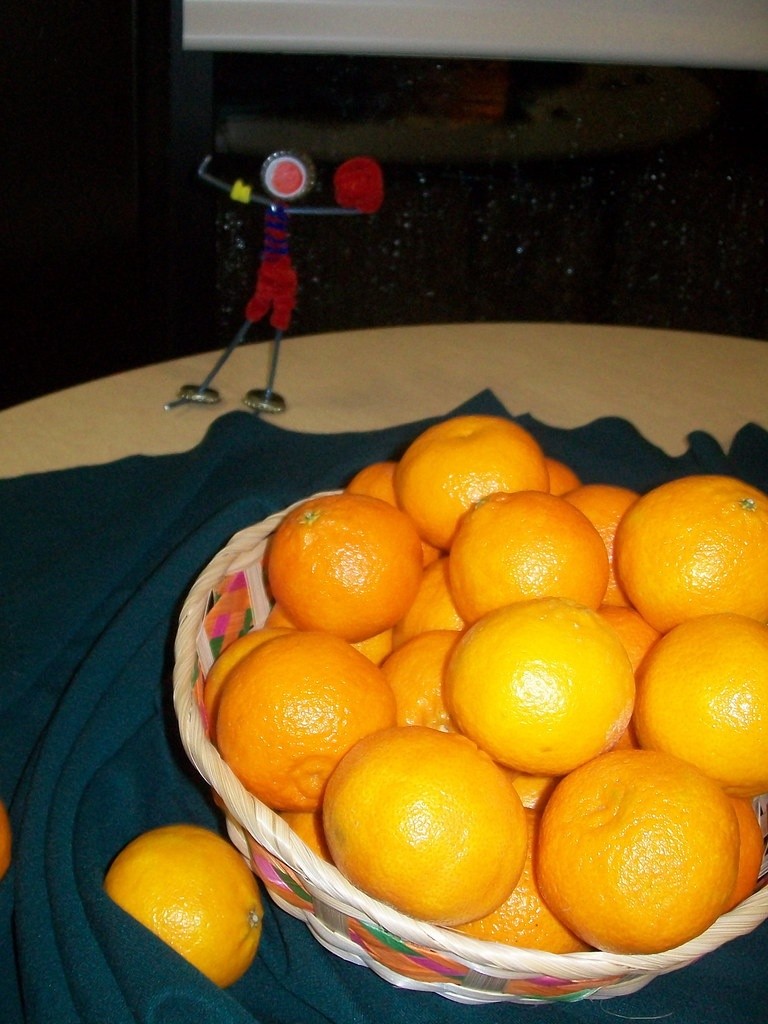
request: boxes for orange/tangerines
[104,825,264,988]
[0,800,12,880]
[202,415,768,954]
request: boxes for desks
[2,325,768,1024]
